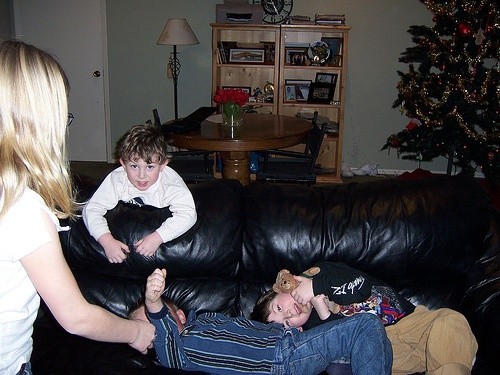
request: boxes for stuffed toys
[273,267,341,314]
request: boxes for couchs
[30,178,500,375]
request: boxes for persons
[252,260,479,375]
[81,125,197,263]
[0,40,156,375]
[286,87,291,96]
[128,268,393,375]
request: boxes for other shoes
[342,165,354,177]
[352,164,377,176]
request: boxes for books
[225,12,254,24]
[285,16,316,25]
[316,14,346,26]
[217,41,238,64]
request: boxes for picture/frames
[321,37,343,67]
[285,72,339,104]
[228,47,266,65]
[222,86,251,95]
[285,47,309,66]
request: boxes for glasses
[67,113,75,126]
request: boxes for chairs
[152,109,328,185]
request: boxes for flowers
[212,88,250,134]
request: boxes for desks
[164,115,313,184]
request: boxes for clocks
[253,0,293,24]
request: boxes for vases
[221,100,241,141]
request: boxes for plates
[308,41,330,63]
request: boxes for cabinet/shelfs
[209,23,352,184]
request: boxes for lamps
[157,18,201,129]
